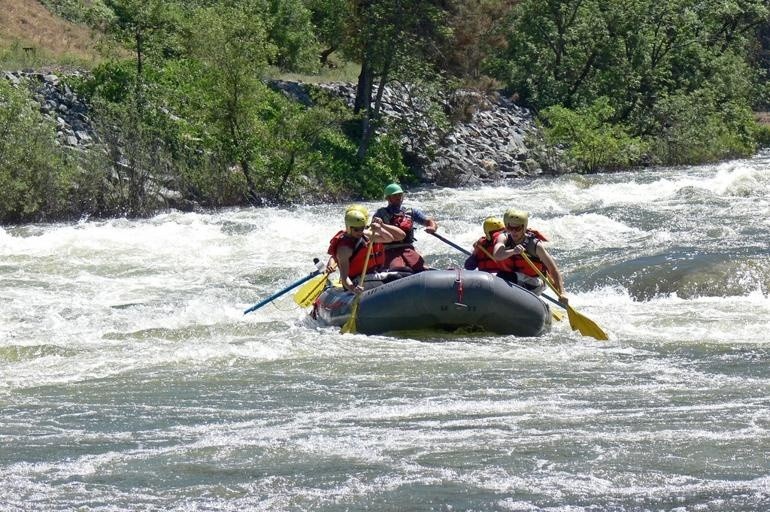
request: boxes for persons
[492,207,564,302]
[326,205,412,276]
[336,210,401,294]
[465,216,506,275]
[369,184,437,272]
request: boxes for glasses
[506,224,524,232]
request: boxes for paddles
[292,227,372,309]
[521,251,609,339]
[478,243,564,322]
[339,230,377,335]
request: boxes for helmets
[345,206,368,235]
[383,184,403,199]
[503,209,528,234]
[483,218,504,240]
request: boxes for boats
[312,266,554,339]
